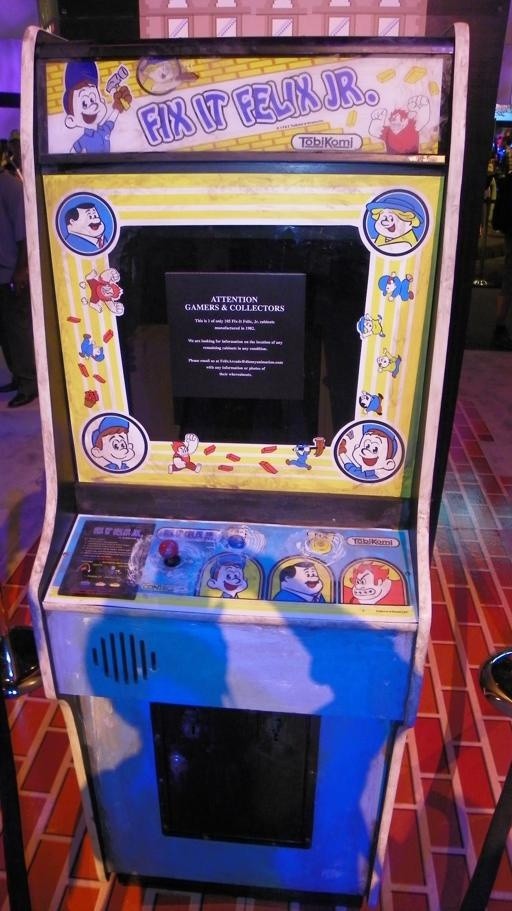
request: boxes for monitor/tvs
[36,160,448,499]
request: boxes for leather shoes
[0,383,19,393]
[8,392,37,407]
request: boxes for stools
[456,645,512,911]
[0,625,43,911]
